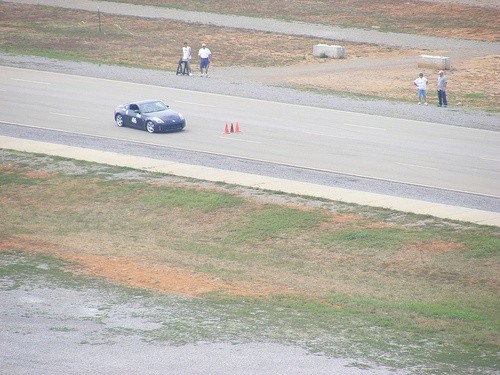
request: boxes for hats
[437,71,444,74]
[202,44,206,46]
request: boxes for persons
[413,72,429,106]
[198,44,211,77]
[180,42,192,76]
[436,71,448,108]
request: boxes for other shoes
[444,105,447,107]
[424,102,428,106]
[438,104,441,107]
[201,74,208,77]
[418,102,421,105]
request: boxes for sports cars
[114,99,186,133]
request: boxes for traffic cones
[224,123,230,134]
[235,122,240,132]
[230,123,234,132]
